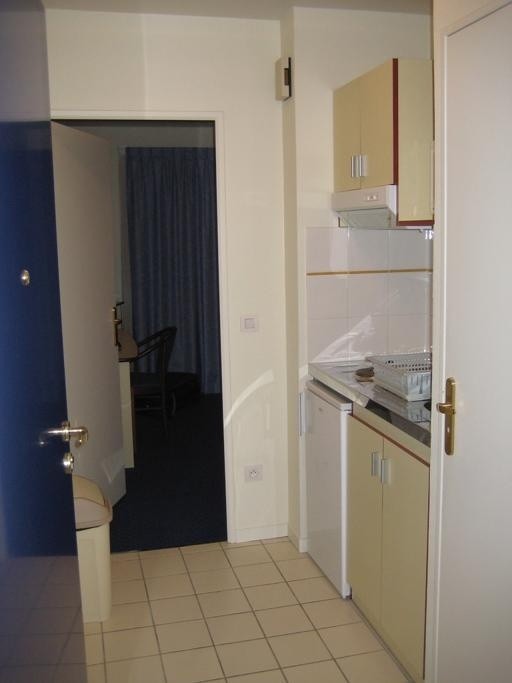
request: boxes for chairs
[129,325,178,439]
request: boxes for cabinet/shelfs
[346,414,430,678]
[332,57,433,226]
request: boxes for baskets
[364,351,432,402]
